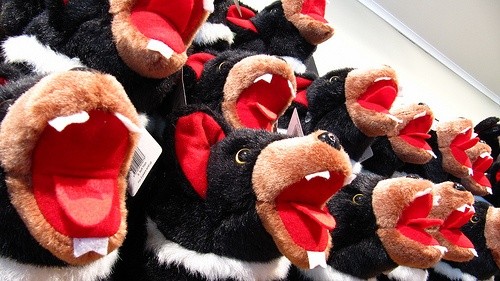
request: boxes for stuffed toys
[0,0,500,281]
